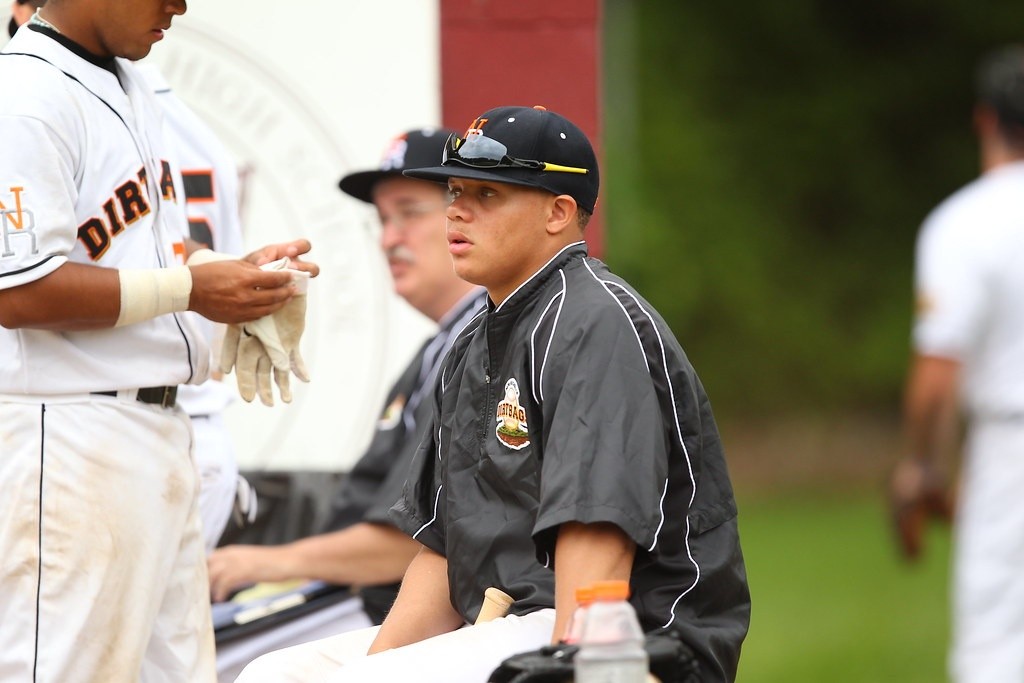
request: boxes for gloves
[218,254,313,405]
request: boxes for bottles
[563,580,649,683]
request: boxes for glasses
[440,132,589,174]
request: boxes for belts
[98,386,179,407]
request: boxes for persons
[232,106,752,683]
[888,43,1024,683]
[0,0,488,683]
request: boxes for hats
[402,105,600,215]
[340,131,460,203]
